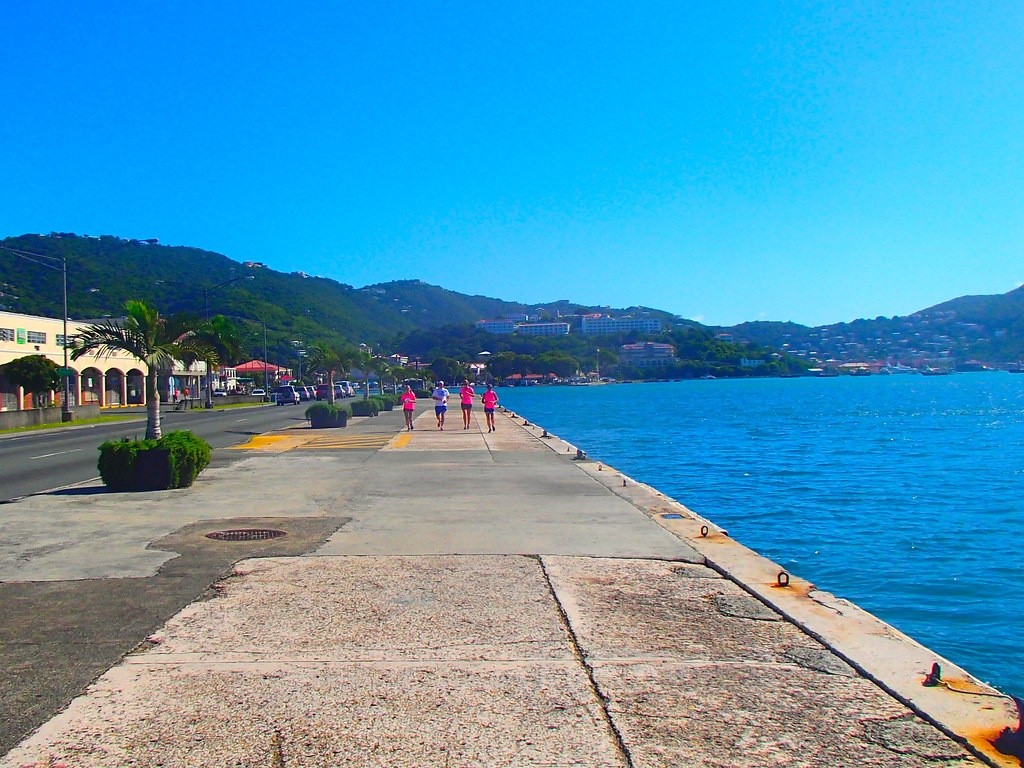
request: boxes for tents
[235,359,279,388]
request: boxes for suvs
[278,385,301,405]
[316,384,337,402]
[334,385,346,399]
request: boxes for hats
[439,381,444,384]
[487,384,493,388]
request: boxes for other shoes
[467,425,469,429]
[488,428,491,433]
[411,426,414,429]
[407,428,410,431]
[464,425,466,429]
[441,426,444,430]
[438,422,440,427]
[492,426,495,431]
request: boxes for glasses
[463,384,467,385]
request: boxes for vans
[335,381,353,398]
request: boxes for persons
[174,387,188,400]
[400,385,416,432]
[482,384,499,433]
[432,381,450,430]
[459,379,475,430]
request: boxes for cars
[306,386,316,400]
[213,388,227,397]
[297,386,311,401]
[250,389,266,396]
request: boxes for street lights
[62,238,160,424]
[263,321,292,402]
[204,276,255,409]
[278,333,302,387]
